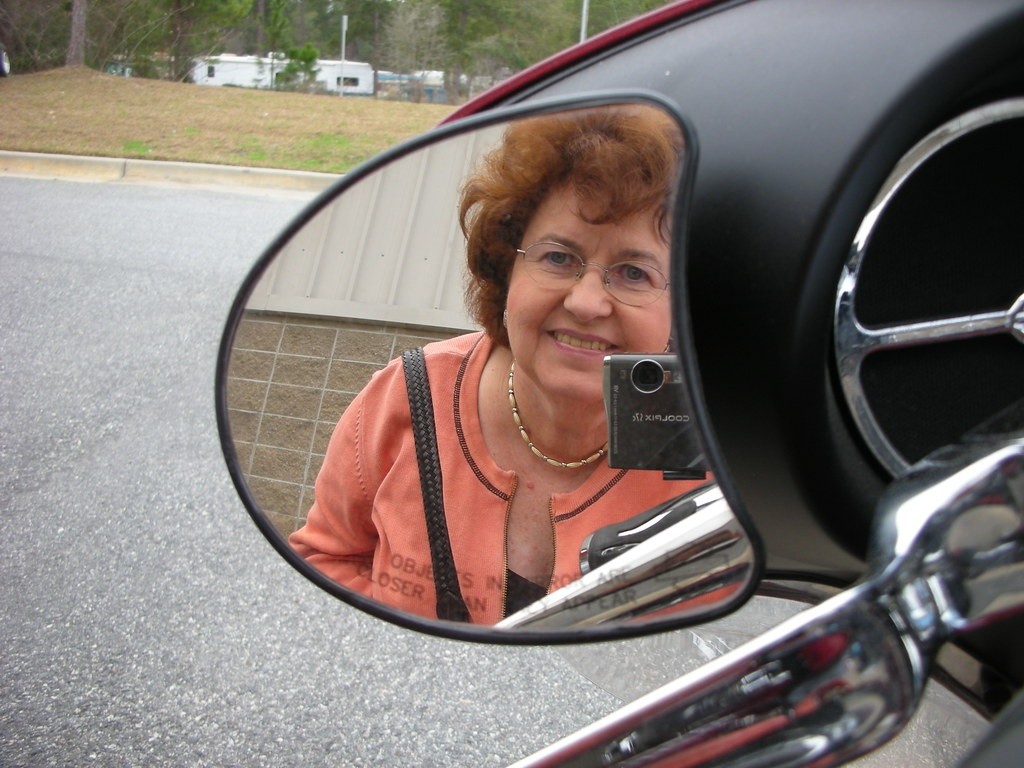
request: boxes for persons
[286,102,744,626]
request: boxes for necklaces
[508,359,610,470]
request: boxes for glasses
[516,243,669,308]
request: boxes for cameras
[602,350,713,479]
